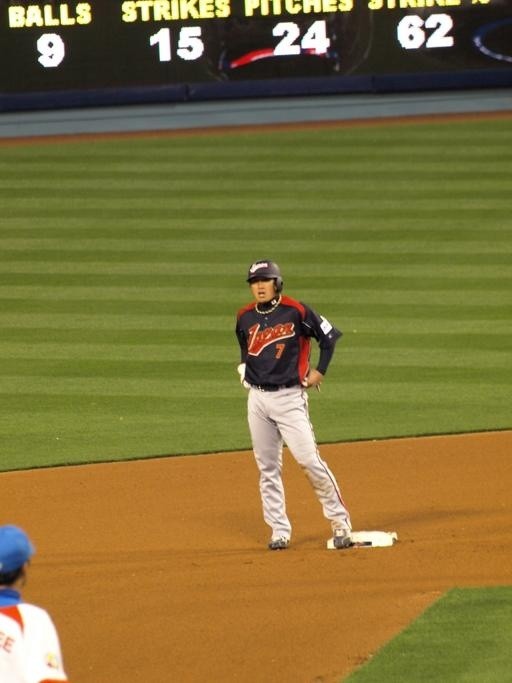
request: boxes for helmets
[0,526,34,573]
[246,260,284,292]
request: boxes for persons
[0,525,71,683]
[236,260,352,550]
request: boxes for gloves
[303,376,322,393]
[236,363,250,389]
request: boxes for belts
[251,379,298,392]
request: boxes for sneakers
[269,536,290,549]
[332,528,352,548]
